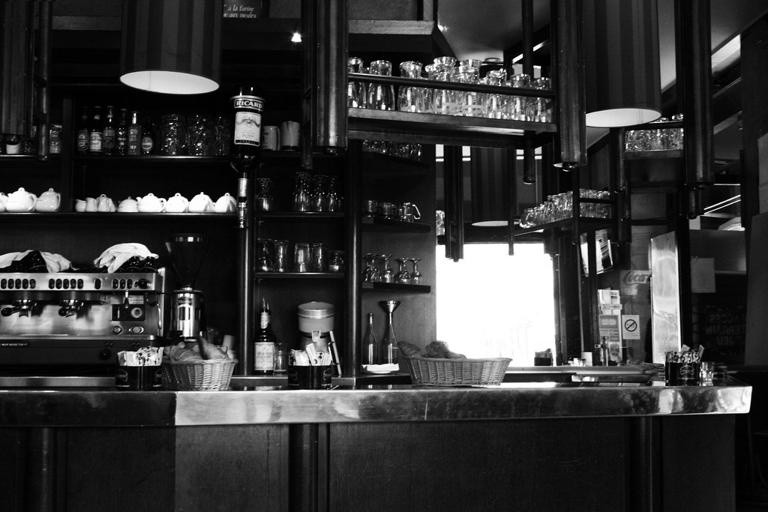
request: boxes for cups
[273,343,288,374]
[75,195,117,212]
[263,120,300,150]
[362,198,421,224]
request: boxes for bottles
[362,312,400,364]
[346,55,553,124]
[255,297,275,376]
[593,335,610,367]
[76,104,140,155]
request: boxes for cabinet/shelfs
[0,0,435,384]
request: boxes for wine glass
[362,253,424,284]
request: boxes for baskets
[162,357,240,391]
[402,353,512,386]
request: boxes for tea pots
[118,193,237,213]
[0,187,62,213]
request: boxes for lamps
[580,2,664,129]
